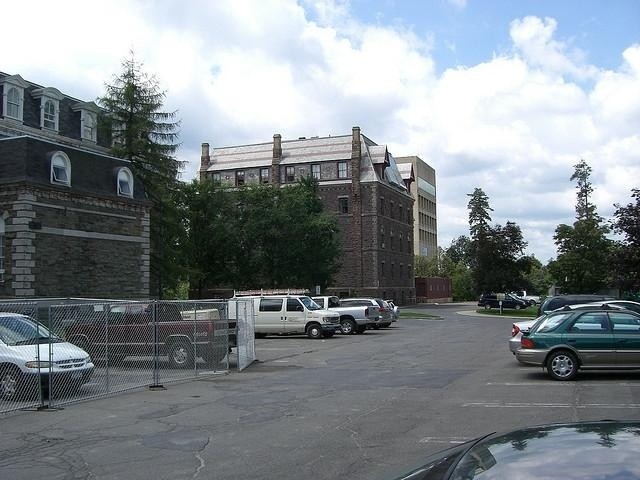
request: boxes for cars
[392,419,640,479]
[509,295,640,380]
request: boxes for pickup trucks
[66,301,237,369]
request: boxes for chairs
[600,318,614,332]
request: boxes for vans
[0,313,95,399]
[478,291,542,310]
[228,294,400,338]
